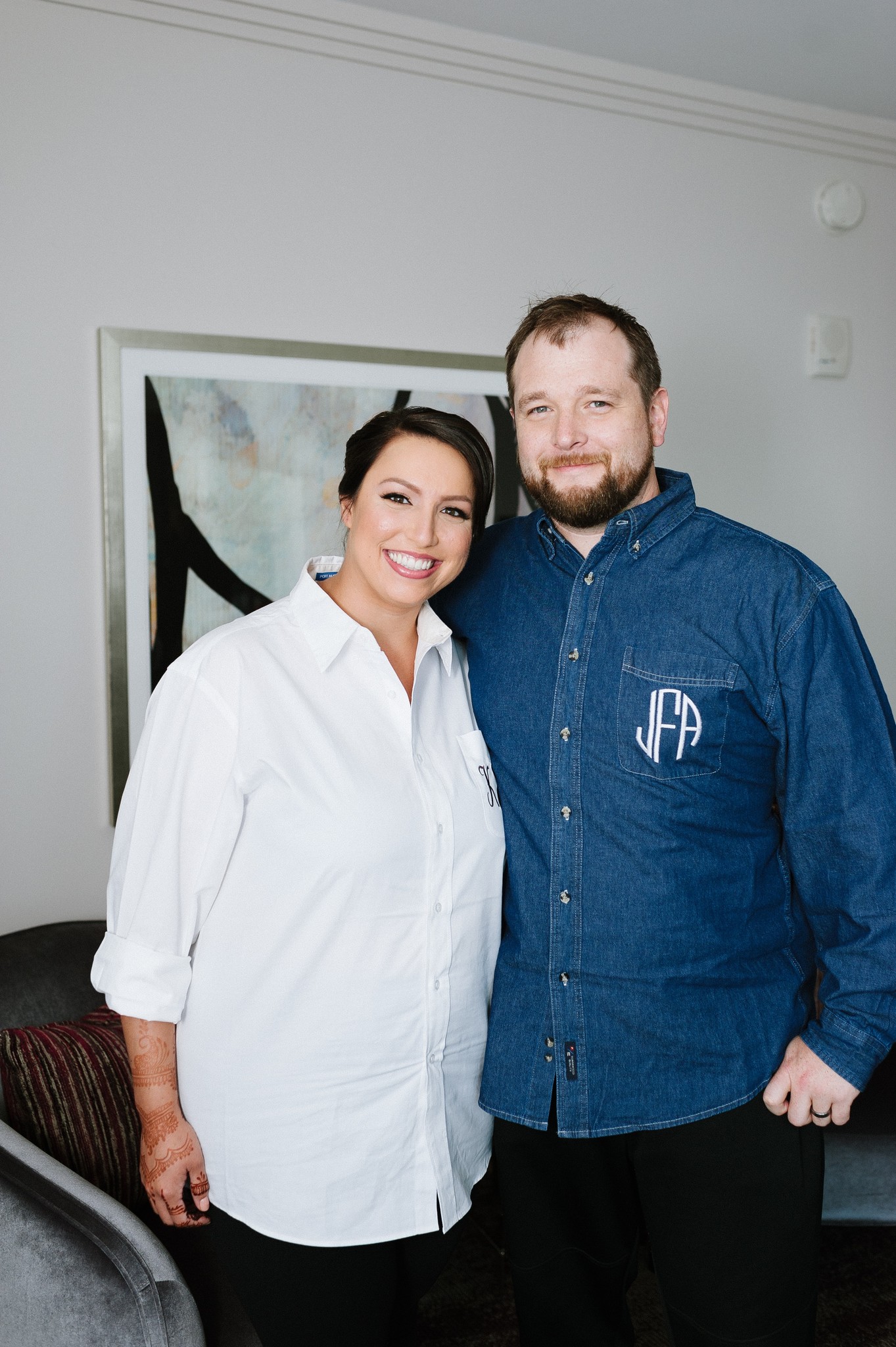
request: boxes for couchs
[3,921,896,1347]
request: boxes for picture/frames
[96,327,541,833]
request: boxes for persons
[423,296,895,1347]
[90,405,508,1347]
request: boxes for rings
[810,1105,832,1118]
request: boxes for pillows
[0,1006,154,1203]
